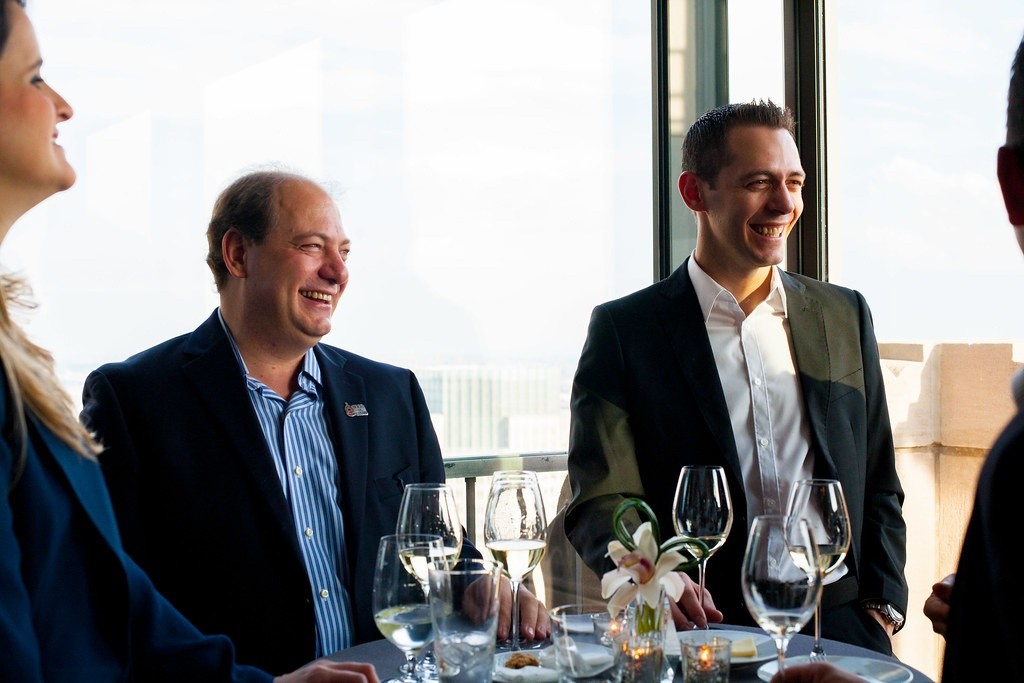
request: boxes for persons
[923,39,1024,683]
[77,172,551,676]
[0,0,381,683]
[562,98,909,658]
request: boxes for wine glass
[742,479,850,683]
[373,484,463,683]
[672,465,733,630]
[483,471,549,652]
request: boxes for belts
[753,575,859,609]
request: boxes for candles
[591,611,734,683]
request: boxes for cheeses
[729,636,759,656]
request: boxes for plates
[675,630,787,663]
[756,655,914,683]
[491,649,561,683]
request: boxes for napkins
[495,663,558,683]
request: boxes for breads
[505,652,540,668]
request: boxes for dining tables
[326,622,934,683]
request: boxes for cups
[548,603,634,683]
[428,559,503,683]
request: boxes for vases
[624,595,671,647]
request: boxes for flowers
[598,498,711,634]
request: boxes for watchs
[862,603,904,630]
